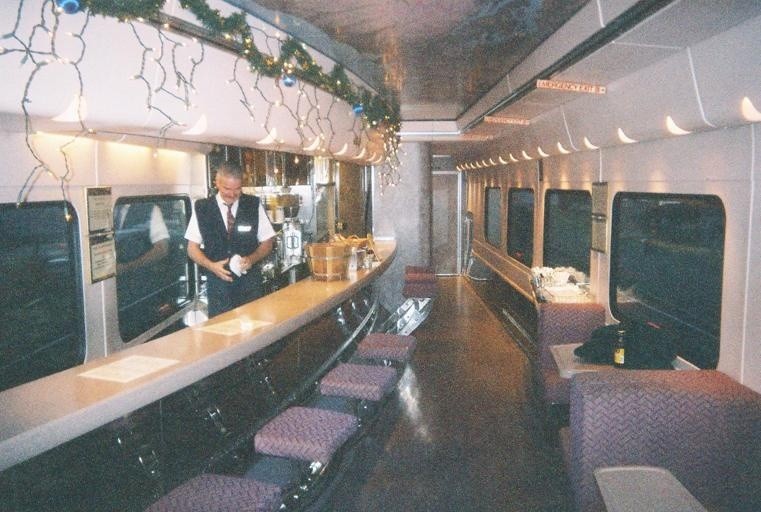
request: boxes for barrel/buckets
[304,242,352,281]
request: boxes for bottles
[612,323,630,368]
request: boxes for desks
[594,466,708,512]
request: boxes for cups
[265,210,285,222]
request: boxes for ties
[114,206,122,229]
[223,203,235,241]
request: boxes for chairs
[536,301,607,405]
[558,372,760,512]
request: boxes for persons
[183,161,278,320]
[113,201,172,343]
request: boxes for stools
[357,332,417,363]
[145,472,281,512]
[254,405,357,462]
[321,362,398,403]
[401,281,438,301]
[406,264,437,280]
